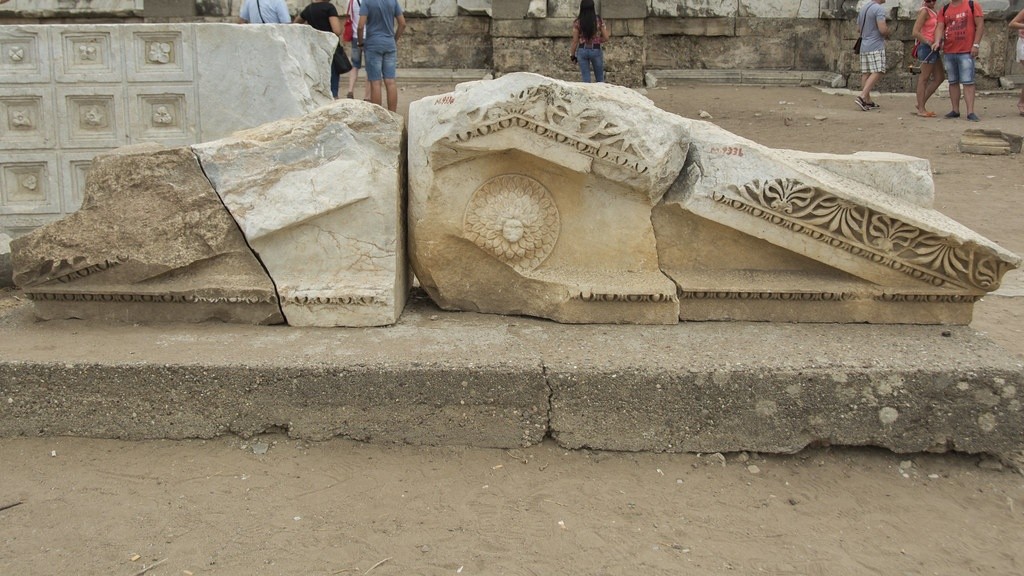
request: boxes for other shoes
[867,102,879,109]
[942,111,960,119]
[1017,102,1024,115]
[967,113,978,122]
[347,91,353,100]
[855,96,869,111]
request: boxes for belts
[579,42,601,49]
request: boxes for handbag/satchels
[853,36,861,55]
[333,46,352,73]
[344,0,353,41]
[912,42,919,58]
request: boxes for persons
[913,0,1024,122]
[569,0,609,83]
[239,0,406,113]
[855,0,893,111]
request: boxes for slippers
[916,105,938,117]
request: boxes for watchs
[357,41,364,47]
[972,43,980,48]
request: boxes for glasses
[926,0,936,3]
